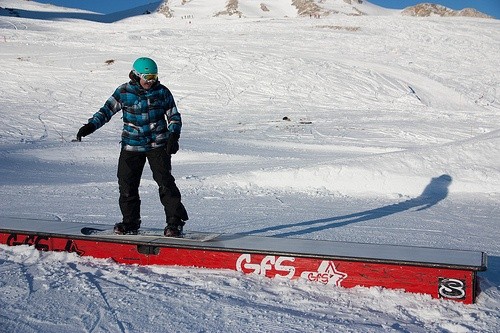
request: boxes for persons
[76,57,189,238]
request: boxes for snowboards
[79,226,222,243]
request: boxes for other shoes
[114,217,141,230]
[164,217,186,236]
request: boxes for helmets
[133,56,157,73]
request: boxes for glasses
[133,70,157,81]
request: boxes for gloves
[167,132,180,154]
[77,121,96,141]
